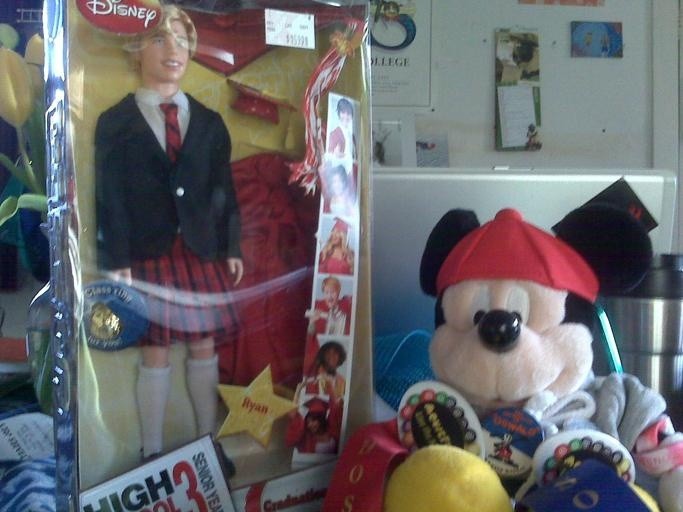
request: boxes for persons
[306,341,346,395]
[328,99,358,157]
[323,164,360,215]
[297,395,344,457]
[312,278,350,335]
[317,217,355,276]
[96,6,244,492]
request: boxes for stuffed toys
[370,202,683,512]
